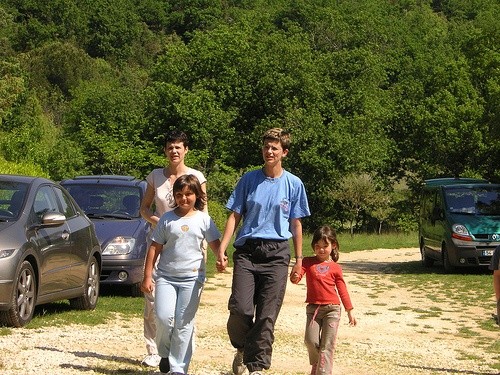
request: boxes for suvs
[59,174,156,298]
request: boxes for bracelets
[294,255,304,259]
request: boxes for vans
[417,172,500,274]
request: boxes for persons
[489,244,500,326]
[292,225,356,375]
[142,174,227,375]
[217,128,311,375]
[139,134,208,368]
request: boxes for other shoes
[159,357,170,373]
[141,355,161,368]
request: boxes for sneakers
[232,349,246,375]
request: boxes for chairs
[123,194,141,214]
[8,190,24,215]
[87,196,106,214]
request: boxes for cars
[0,174,102,328]
